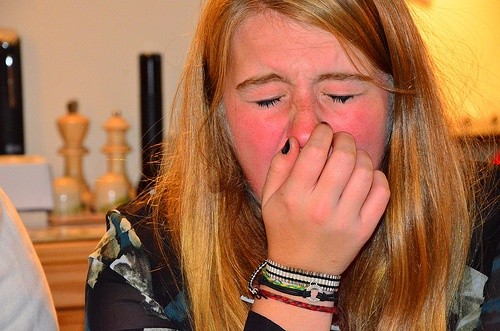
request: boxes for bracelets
[239,260,341,315]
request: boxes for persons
[84,0,500,331]
[0,188,59,331]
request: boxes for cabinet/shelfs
[16,211,109,331]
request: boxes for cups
[97,178,129,213]
[53,177,80,217]
